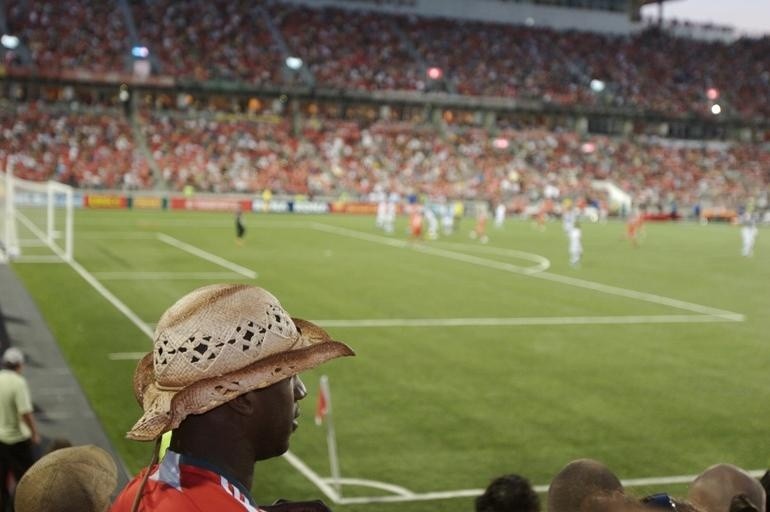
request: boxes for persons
[684,461,767,511]
[0,0,769,263]
[545,456,625,511]
[0,346,41,509]
[471,472,542,510]
[103,276,355,512]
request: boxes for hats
[668,463,769,511]
[2,346,25,365]
[124,280,358,443]
